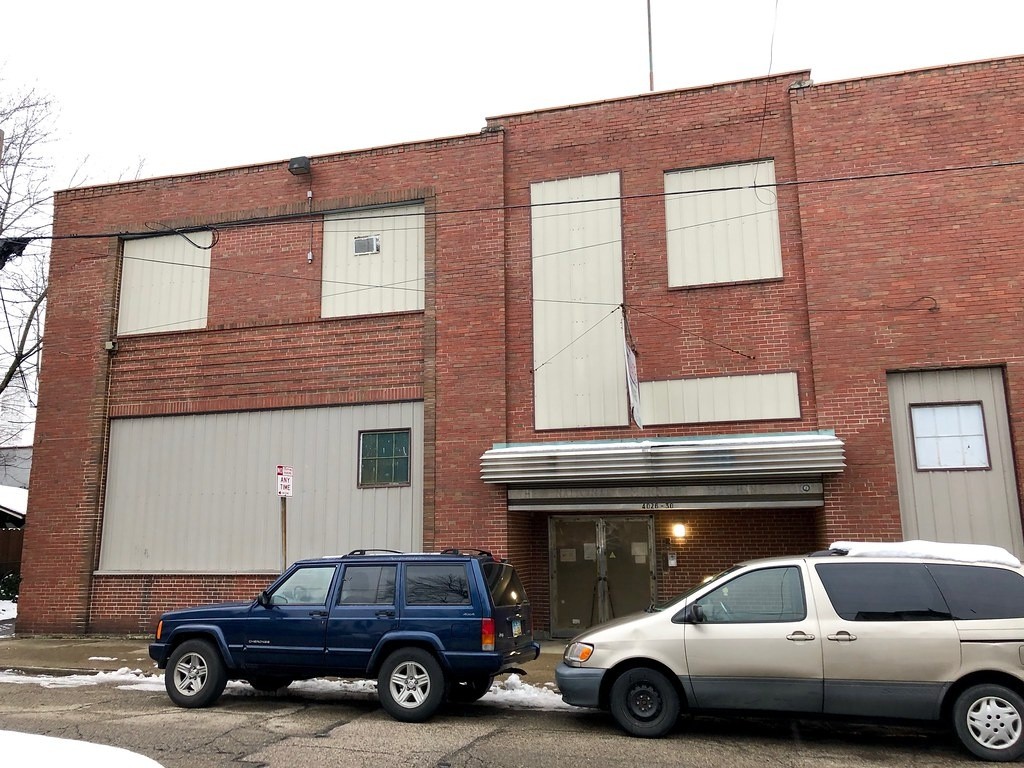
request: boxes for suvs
[553,538,1024,764]
[147,545,542,723]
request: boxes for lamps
[288,156,311,177]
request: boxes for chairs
[344,572,370,603]
